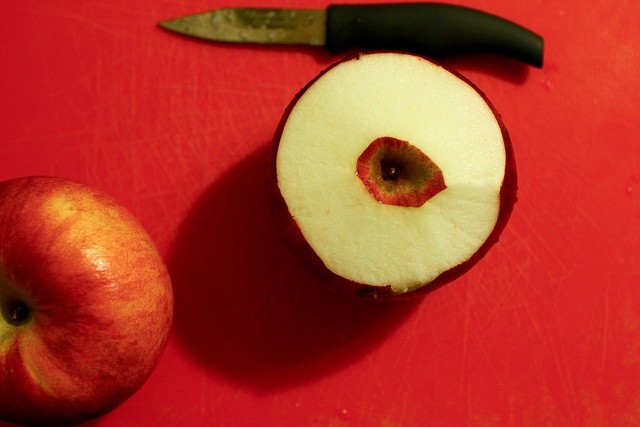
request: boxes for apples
[0,176,176,427]
[269,49,520,299]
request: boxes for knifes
[161,4,542,67]
